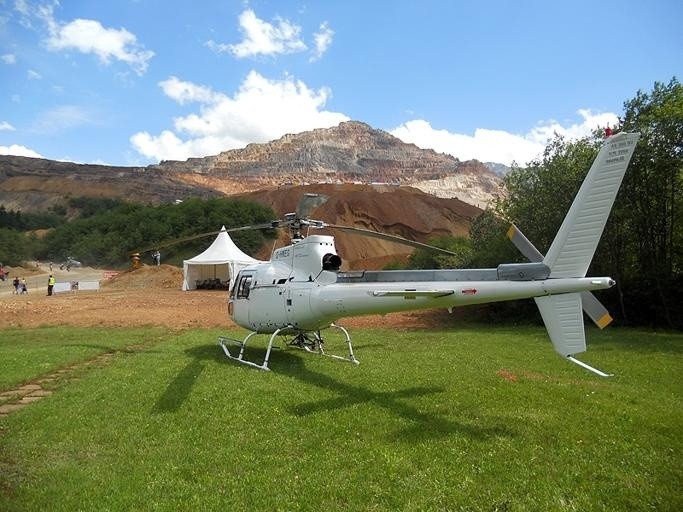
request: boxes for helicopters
[128,131,644,379]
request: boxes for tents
[180,224,259,292]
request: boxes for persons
[0,265,28,296]
[154,250,160,264]
[150,251,155,264]
[32,260,74,273]
[45,274,55,296]
